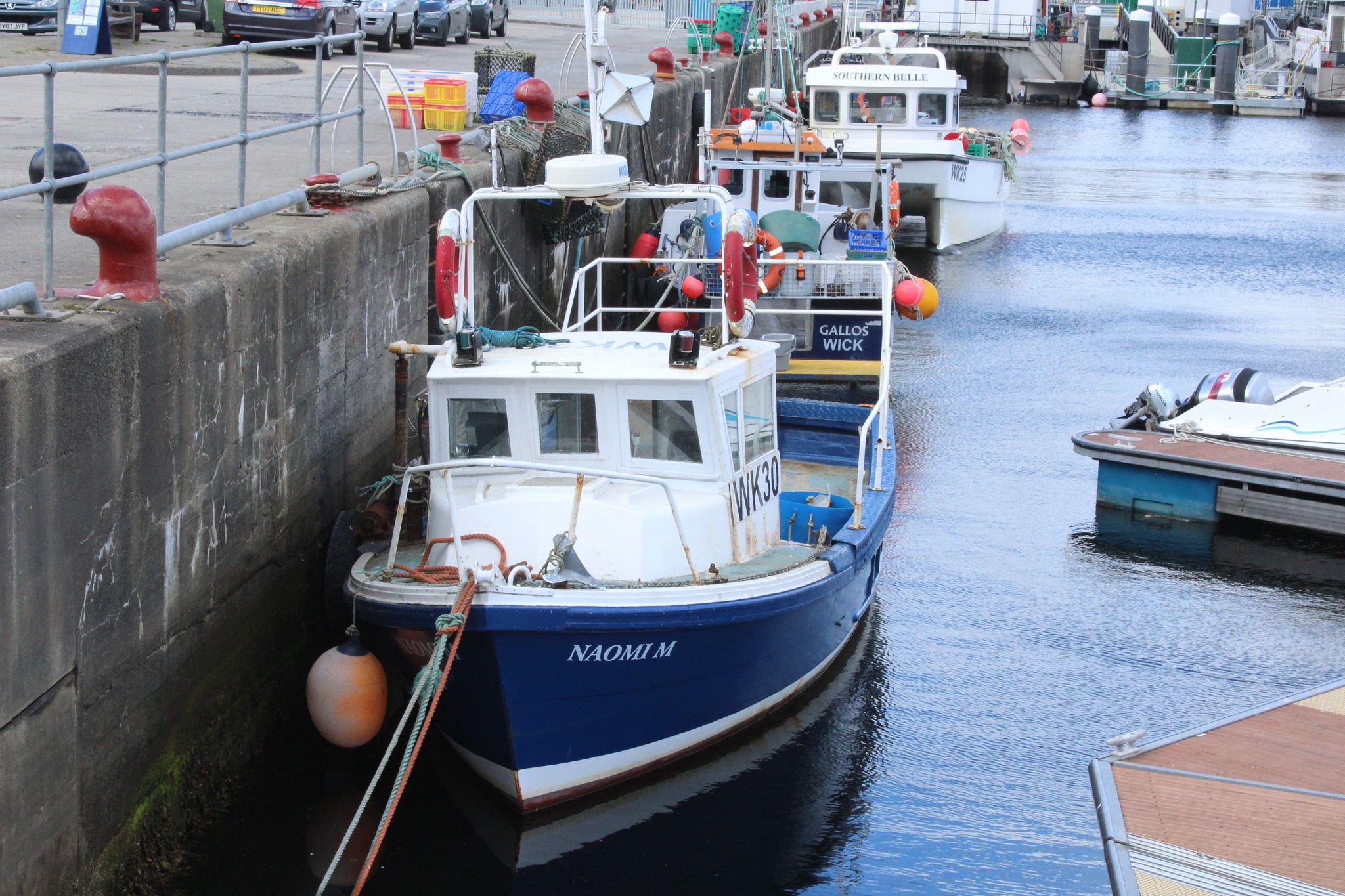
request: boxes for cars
[341,0,420,52]
[221,0,365,60]
[110,0,208,31]
[0,0,59,38]
[466,0,509,39]
[417,0,472,47]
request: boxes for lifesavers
[717,229,786,297]
[889,179,900,228]
[723,209,759,338]
[435,208,468,335]
[892,96,897,106]
[857,93,887,109]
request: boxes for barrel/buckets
[760,333,797,371]
[704,209,758,291]
[759,210,822,253]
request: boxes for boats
[700,14,1017,255]
[299,0,899,815]
